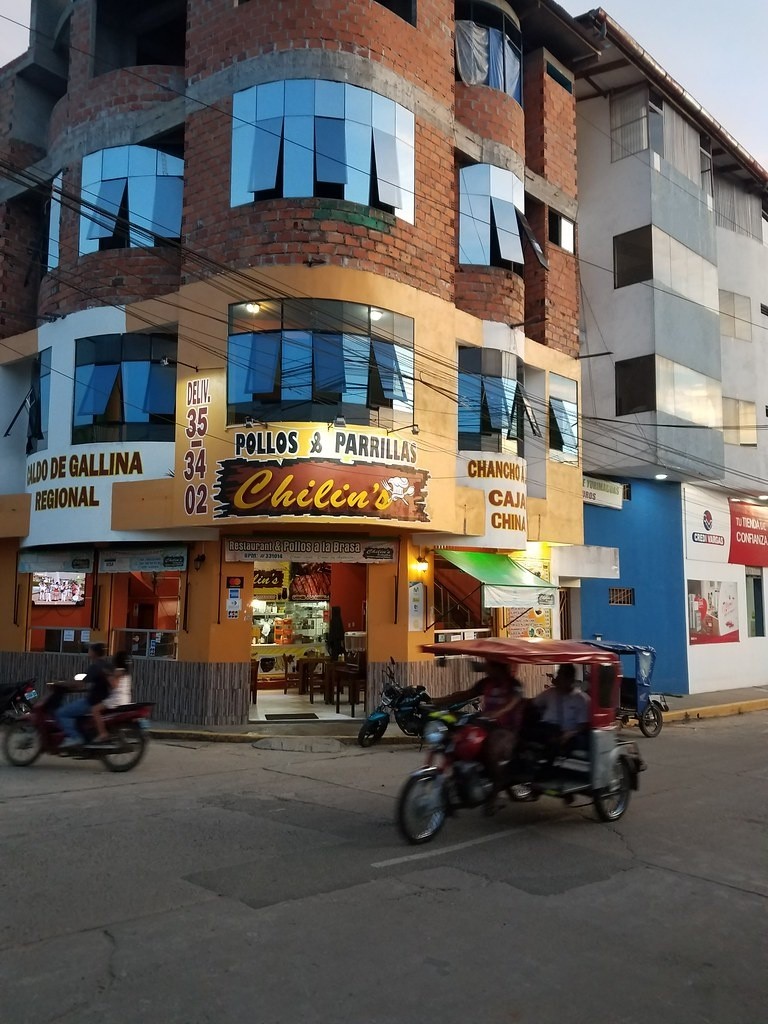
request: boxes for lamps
[326,416,347,429]
[369,309,384,320]
[244,303,260,313]
[160,354,200,372]
[194,554,206,571]
[244,416,270,430]
[388,425,421,436]
[418,556,428,572]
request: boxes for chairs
[283,653,335,705]
[250,659,259,704]
[335,651,366,717]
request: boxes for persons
[54,642,133,748]
[432,661,524,818]
[324,605,348,663]
[39,578,79,602]
[525,663,590,784]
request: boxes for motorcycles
[0,677,37,720]
[396,636,649,844]
[545,637,670,738]
[4,673,157,773]
[358,656,483,752]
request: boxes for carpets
[265,712,320,720]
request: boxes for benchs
[546,725,612,772]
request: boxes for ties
[555,690,565,725]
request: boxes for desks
[298,657,332,695]
[336,668,358,705]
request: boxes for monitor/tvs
[31,572,87,607]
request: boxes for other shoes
[57,735,85,748]
[92,734,112,742]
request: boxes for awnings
[433,549,560,608]
